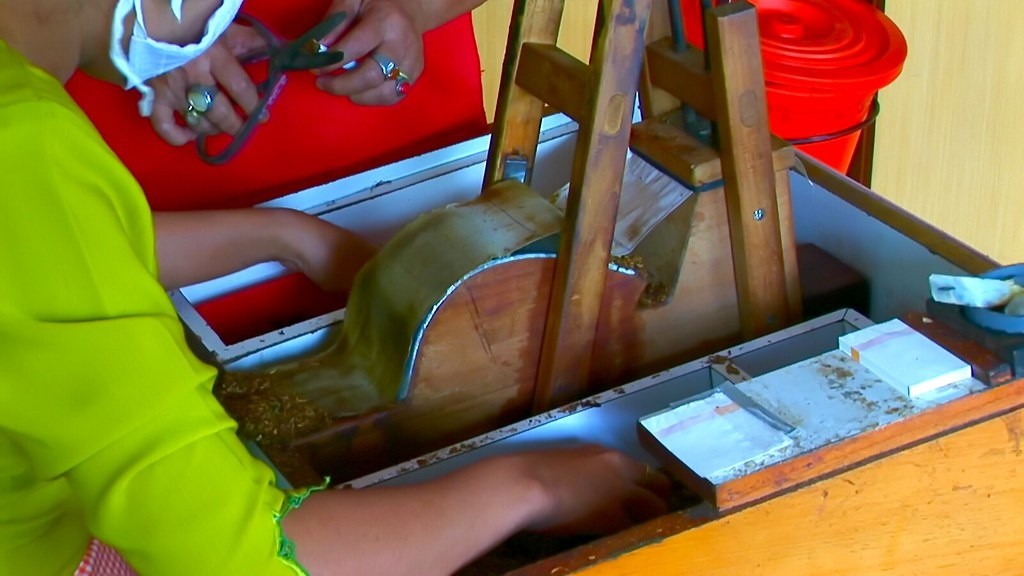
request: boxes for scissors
[197,9,347,166]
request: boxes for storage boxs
[839,318,971,398]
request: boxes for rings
[395,72,412,96]
[184,105,200,128]
[370,49,402,82]
[185,82,221,114]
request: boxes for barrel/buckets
[678,1,908,174]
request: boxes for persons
[71,0,500,206]
[1,0,685,575]
